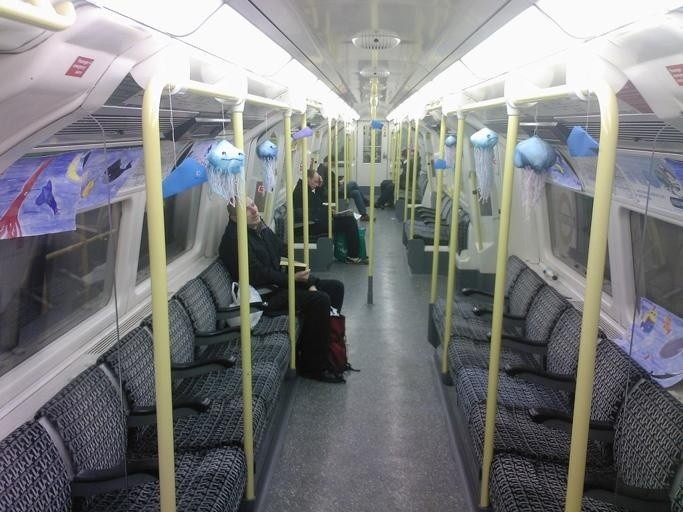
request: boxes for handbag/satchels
[226,280,264,332]
[334,226,369,266]
[327,305,348,373]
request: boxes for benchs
[274,167,352,270]
[390,170,471,275]
[0,257,319,512]
[432,256,683,512]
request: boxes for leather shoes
[299,366,343,382]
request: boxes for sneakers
[360,213,376,221]
[344,255,369,265]
[364,199,393,210]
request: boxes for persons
[316,155,377,221]
[219,196,349,383]
[410,149,421,175]
[294,169,367,264]
[373,148,418,209]
